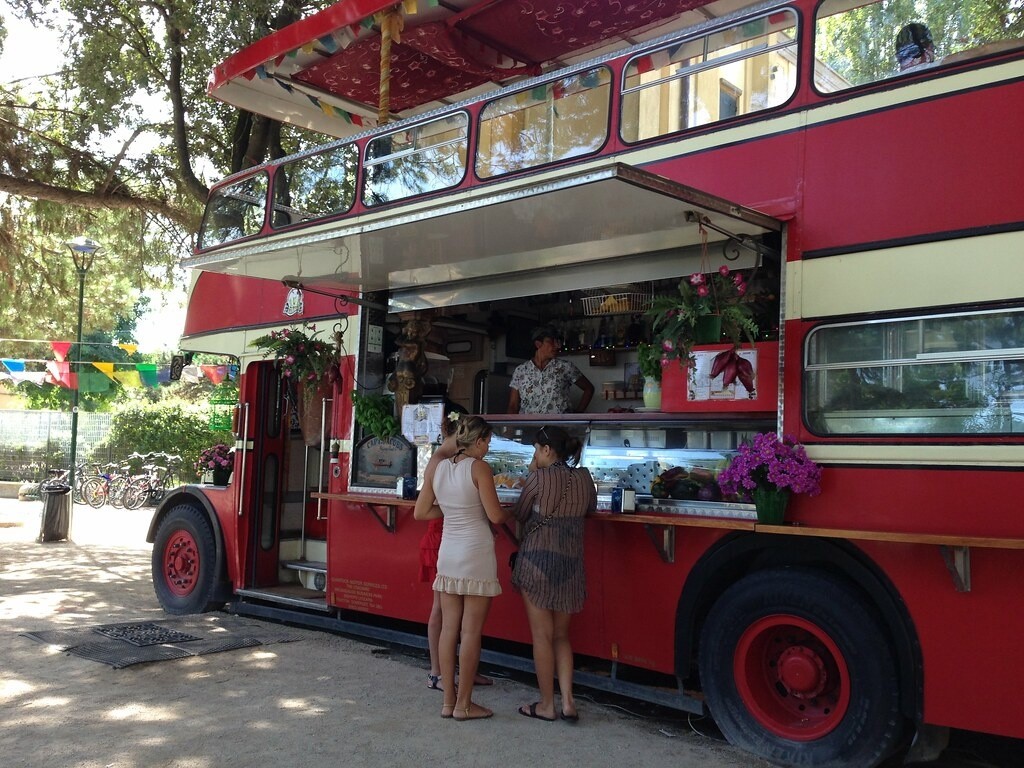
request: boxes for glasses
[539,426,549,439]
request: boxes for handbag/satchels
[508,551,520,572]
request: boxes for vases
[693,313,722,343]
[751,487,791,524]
[213,471,231,486]
[643,377,661,408]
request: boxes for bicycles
[39,452,183,511]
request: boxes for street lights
[63,236,103,486]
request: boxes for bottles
[596,316,625,348]
[627,314,649,347]
[626,377,645,391]
[547,296,582,314]
[611,488,622,513]
[625,489,635,513]
[569,318,595,352]
[551,319,568,352]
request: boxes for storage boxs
[42,483,71,540]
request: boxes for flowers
[718,432,824,501]
[663,266,748,351]
[195,444,234,474]
[256,319,345,394]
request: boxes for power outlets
[369,326,383,353]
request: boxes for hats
[532,325,564,342]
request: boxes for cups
[672,479,693,500]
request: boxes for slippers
[519,702,555,721]
[560,708,579,721]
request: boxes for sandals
[441,702,456,718]
[427,674,459,694]
[458,671,494,685]
[453,703,493,721]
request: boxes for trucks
[146,0,1024,768]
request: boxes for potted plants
[637,342,663,376]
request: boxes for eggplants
[697,483,722,501]
[660,466,684,483]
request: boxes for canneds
[612,487,623,513]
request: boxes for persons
[501,328,595,445]
[895,22,936,74]
[414,403,493,695]
[509,425,597,722]
[430,416,516,722]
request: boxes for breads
[492,474,526,488]
